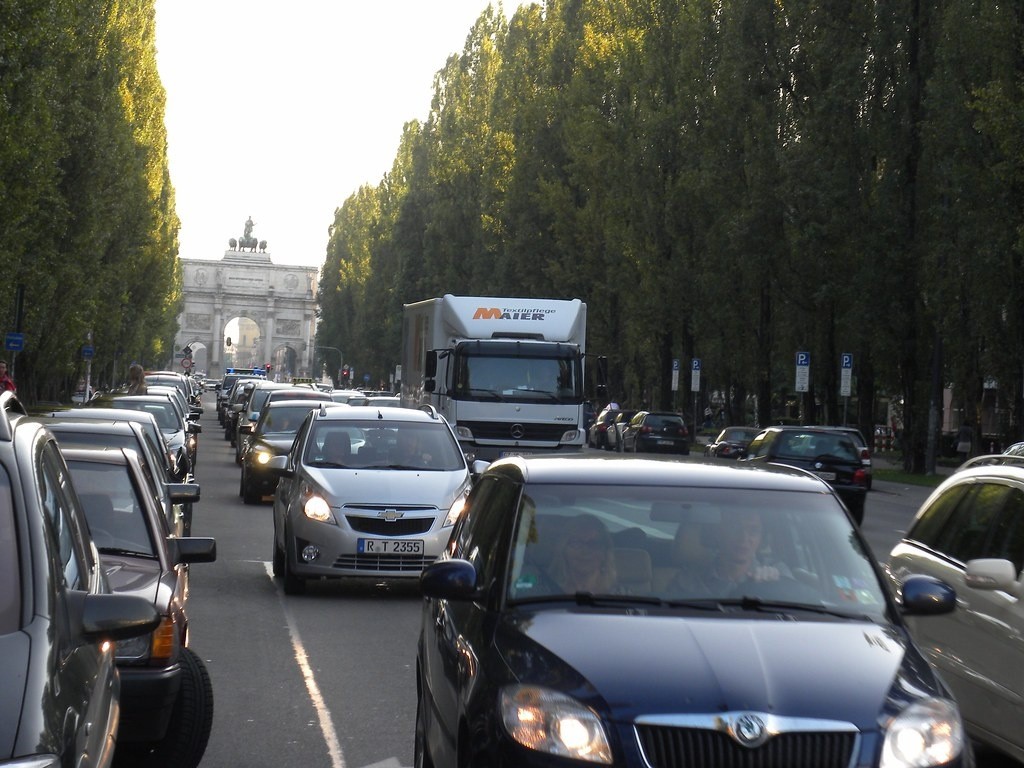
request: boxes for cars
[215,366,401,464]
[737,425,872,528]
[703,427,761,462]
[32,370,218,768]
[582,399,635,451]
[883,444,1024,765]
[616,411,690,456]
[239,401,366,504]
[414,451,975,768]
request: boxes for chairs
[320,430,353,463]
[545,544,652,599]
[656,519,777,601]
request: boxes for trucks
[398,294,609,471]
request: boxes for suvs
[273,405,490,596]
[0,388,163,768]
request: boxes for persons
[524,513,641,594]
[715,404,724,426]
[704,403,713,428]
[393,427,435,467]
[127,365,147,395]
[0,360,17,393]
[954,418,977,464]
[673,503,797,602]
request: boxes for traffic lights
[227,337,231,346]
[267,364,270,373]
[342,370,348,378]
